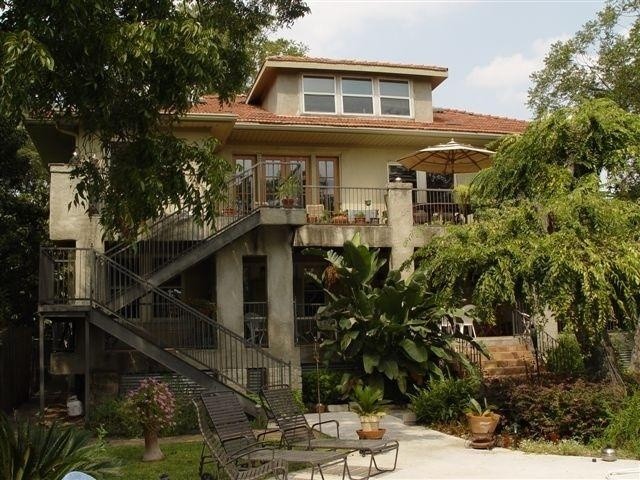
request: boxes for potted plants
[512,381,596,446]
[462,396,503,433]
[349,383,387,441]
[220,192,237,216]
[276,176,301,208]
[184,295,216,320]
[307,209,388,225]
[116,376,175,463]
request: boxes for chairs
[258,383,399,480]
[190,394,288,480]
[439,299,477,338]
[244,312,267,347]
[414,203,464,226]
[200,390,348,479]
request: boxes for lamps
[72,145,79,156]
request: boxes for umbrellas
[394,138,500,191]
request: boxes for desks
[413,202,475,224]
